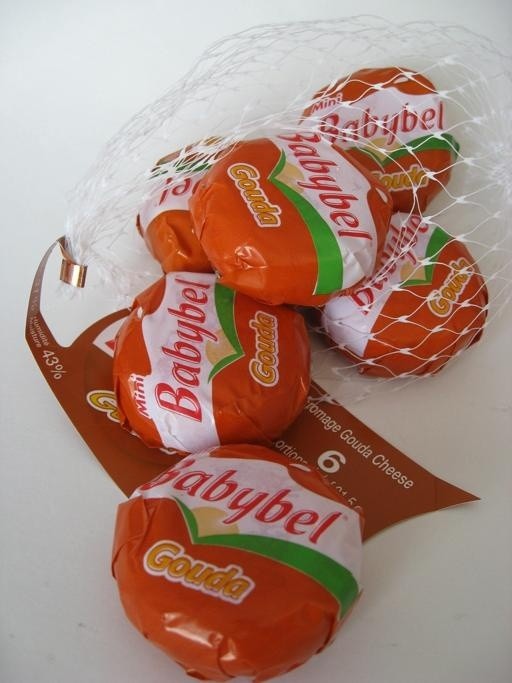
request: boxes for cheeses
[113,271,310,455]
[321,211,488,378]
[137,135,242,276]
[110,444,363,676]
[297,68,458,215]
[189,133,393,306]
